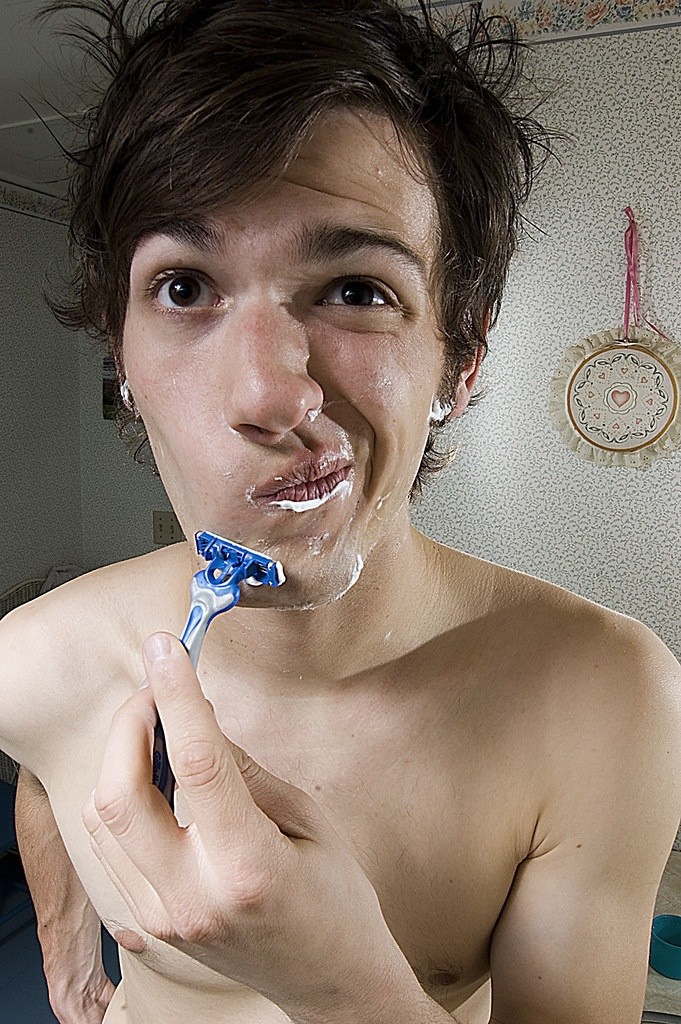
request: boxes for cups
[649,913,681,980]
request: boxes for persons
[0,0,681,1024]
